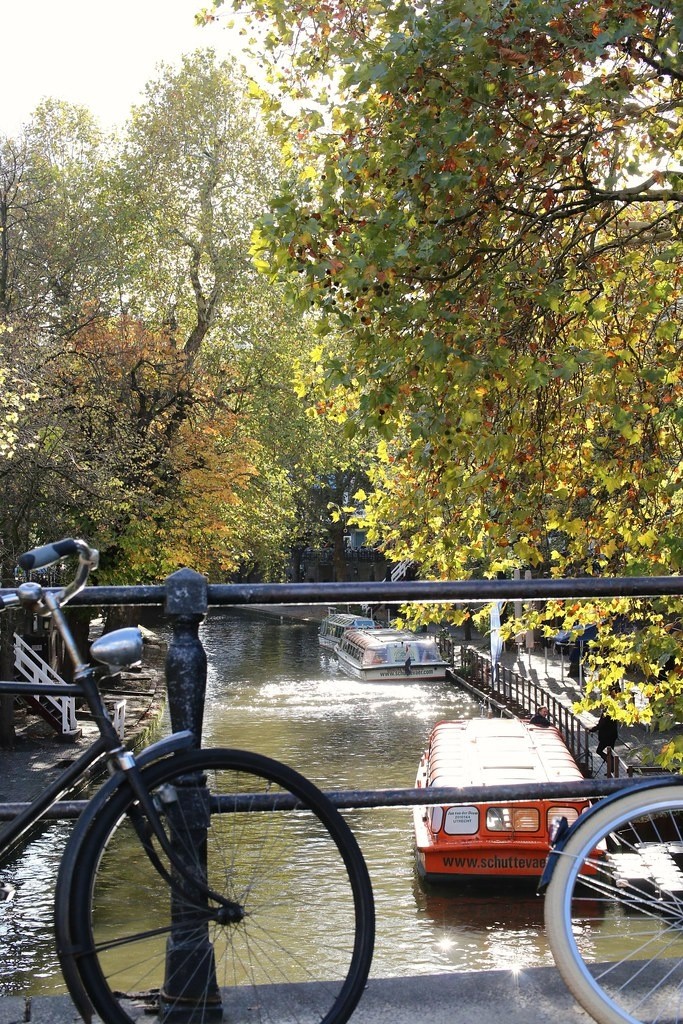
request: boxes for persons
[529,706,553,726]
[405,645,411,675]
[588,701,618,777]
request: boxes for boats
[317,613,382,650]
[334,627,452,682]
[412,717,605,883]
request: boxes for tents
[470,602,683,696]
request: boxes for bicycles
[536,776,682,1023]
[1,534,376,1023]
